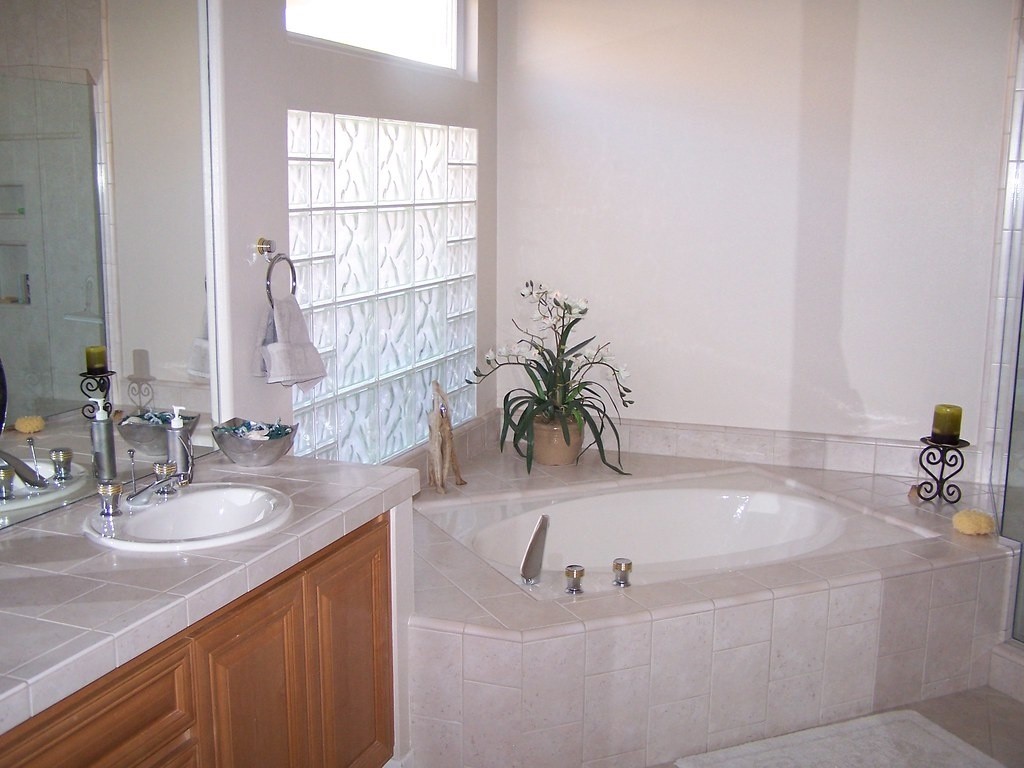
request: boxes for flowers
[463,279,636,479]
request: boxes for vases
[525,412,585,468]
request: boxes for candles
[85,346,108,374]
[929,403,964,443]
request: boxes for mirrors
[0,0,122,431]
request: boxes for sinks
[0,458,87,511]
[82,481,295,552]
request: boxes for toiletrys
[168,406,188,474]
[88,397,115,481]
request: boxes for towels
[254,296,325,387]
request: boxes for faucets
[0,451,49,487]
[126,474,189,505]
[519,515,550,584]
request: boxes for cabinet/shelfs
[0,624,217,768]
[188,508,394,768]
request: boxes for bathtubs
[390,441,1021,767]
